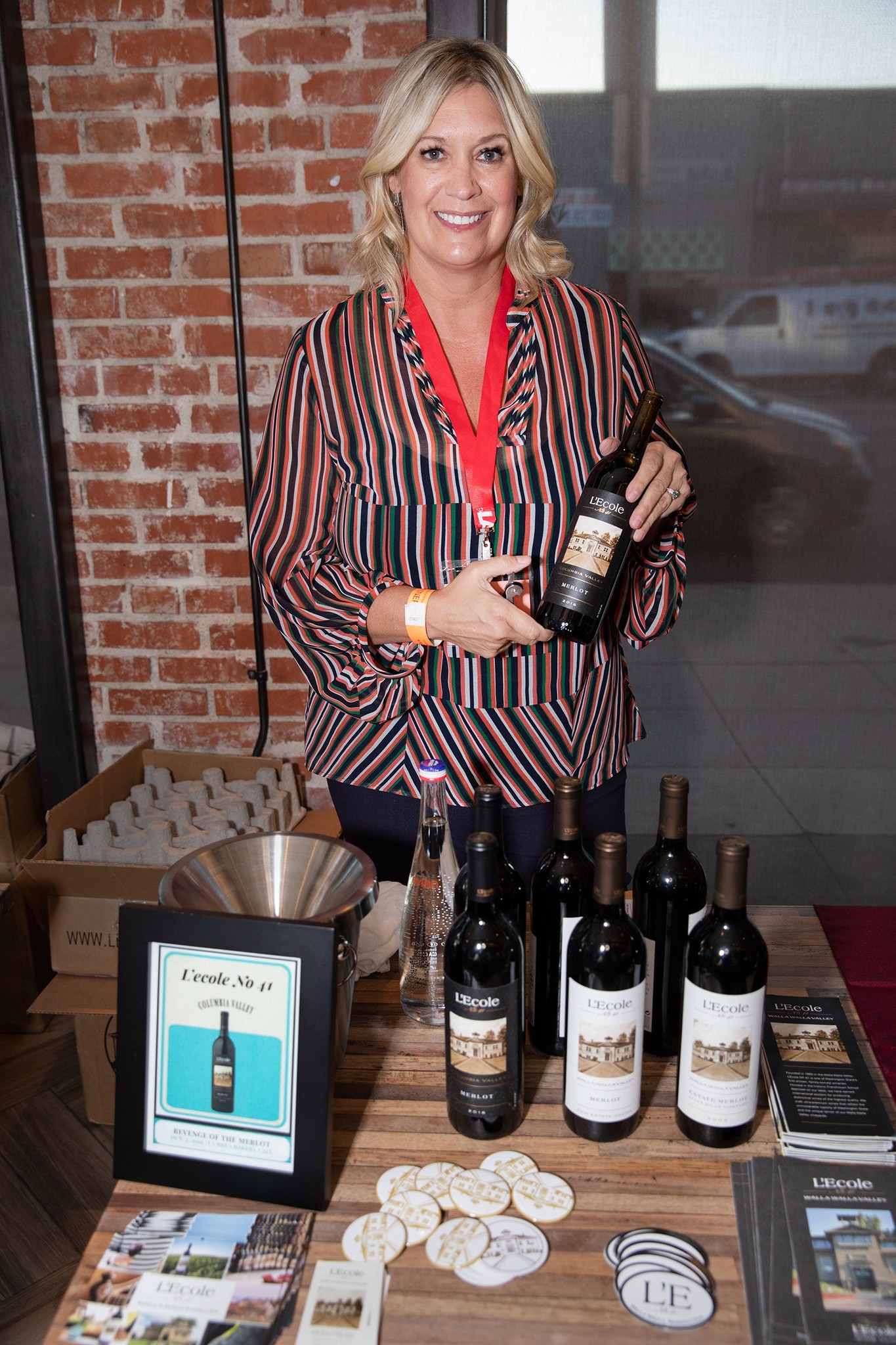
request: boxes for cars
[632,333,866,548]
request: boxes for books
[56,1209,387,1345]
[728,990,896,1345]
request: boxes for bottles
[211,1011,235,1113]
[396,755,774,1148]
[531,391,663,644]
[98,1306,125,1345]
[175,1242,193,1273]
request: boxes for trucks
[677,284,895,394]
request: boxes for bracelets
[404,586,444,647]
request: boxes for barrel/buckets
[160,826,379,1066]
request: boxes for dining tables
[39,902,895,1344]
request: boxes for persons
[240,36,703,894]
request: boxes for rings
[665,487,679,501]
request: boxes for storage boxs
[19,737,341,1125]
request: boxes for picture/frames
[113,902,340,1213]
